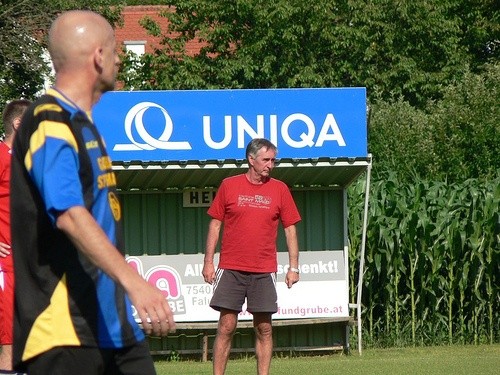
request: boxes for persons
[10,9,177,375]
[0,100,35,372]
[201,138,300,374]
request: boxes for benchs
[123,248,351,364]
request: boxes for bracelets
[288,268,300,273]
